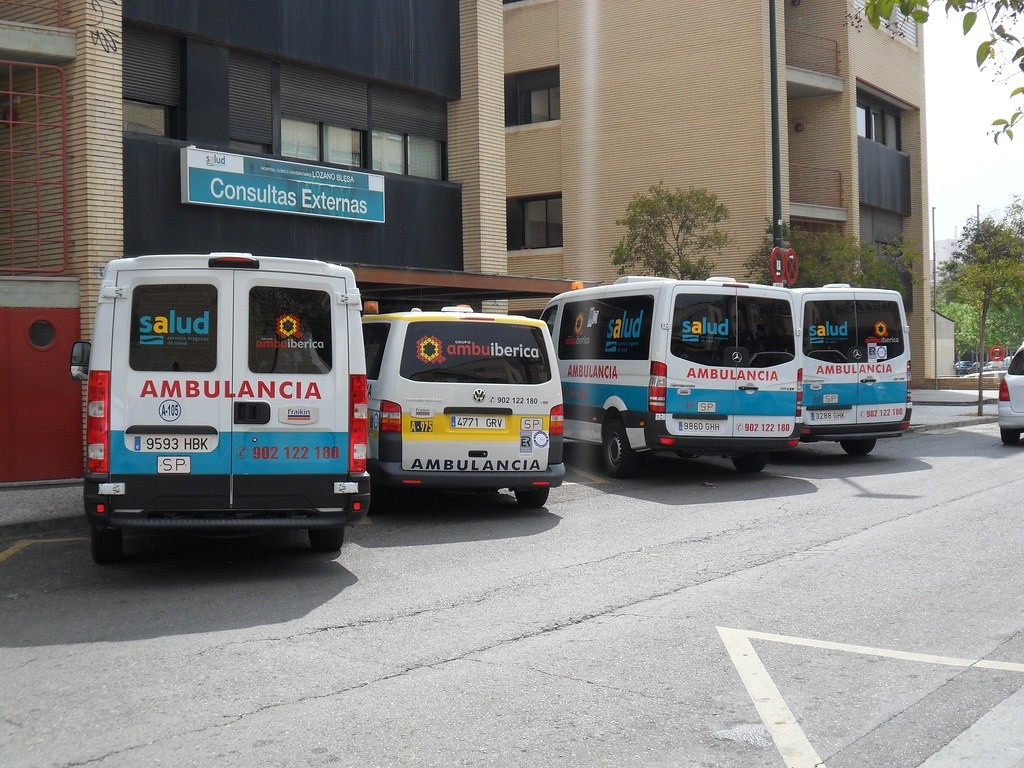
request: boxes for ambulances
[69,252,370,565]
[539,276,804,479]
[363,300,565,508]
[705,277,913,455]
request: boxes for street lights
[977,204,981,227]
[931,207,937,389]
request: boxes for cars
[955,361,972,374]
[983,361,999,371]
[1001,356,1014,368]
[998,341,1024,445]
[965,362,984,373]
